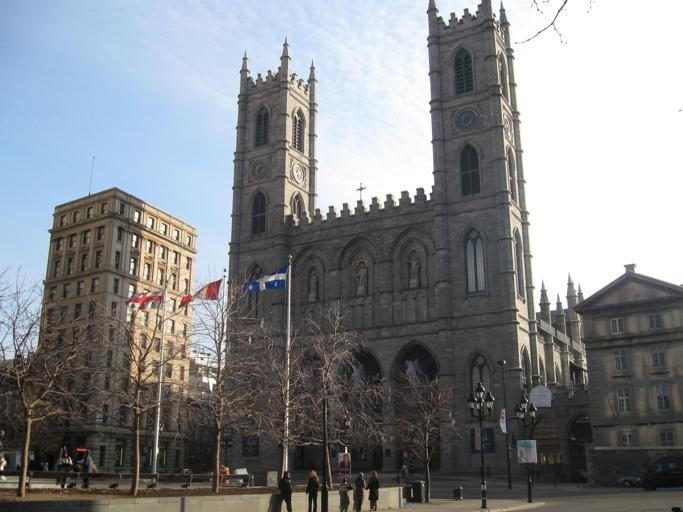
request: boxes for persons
[304,470,320,512]
[55,450,93,488]
[278,470,294,512]
[338,470,380,512]
[16,462,49,472]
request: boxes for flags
[125,263,291,309]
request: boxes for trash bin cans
[267,470,278,488]
[406,480,426,503]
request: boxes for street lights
[513,392,536,502]
[466,382,495,509]
[496,359,508,490]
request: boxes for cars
[616,454,683,491]
